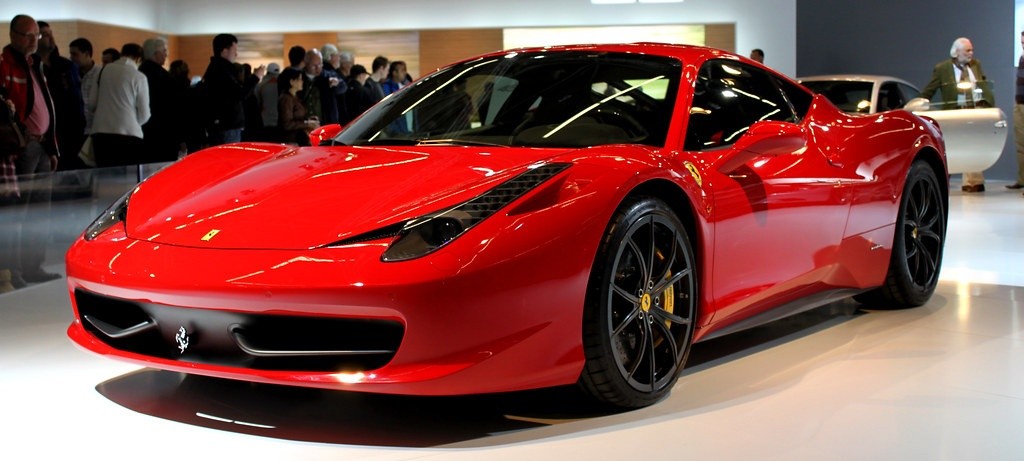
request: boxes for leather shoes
[1005,184,1024,190]
[26,270,61,284]
[962,185,983,193]
[11,275,29,290]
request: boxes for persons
[1006,31,1024,197]
[750,49,764,64]
[165,57,191,165]
[88,40,154,212]
[30,21,83,244]
[102,45,120,64]
[915,38,994,192]
[137,37,178,177]
[252,43,498,147]
[0,15,61,290]
[66,32,104,199]
[1,72,25,291]
[204,32,264,147]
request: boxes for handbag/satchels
[77,135,97,167]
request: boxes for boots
[0,269,14,293]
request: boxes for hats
[349,64,371,78]
[266,63,278,74]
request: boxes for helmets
[320,44,337,61]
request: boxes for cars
[794,74,1009,175]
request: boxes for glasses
[15,31,42,40]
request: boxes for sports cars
[63,42,950,412]
